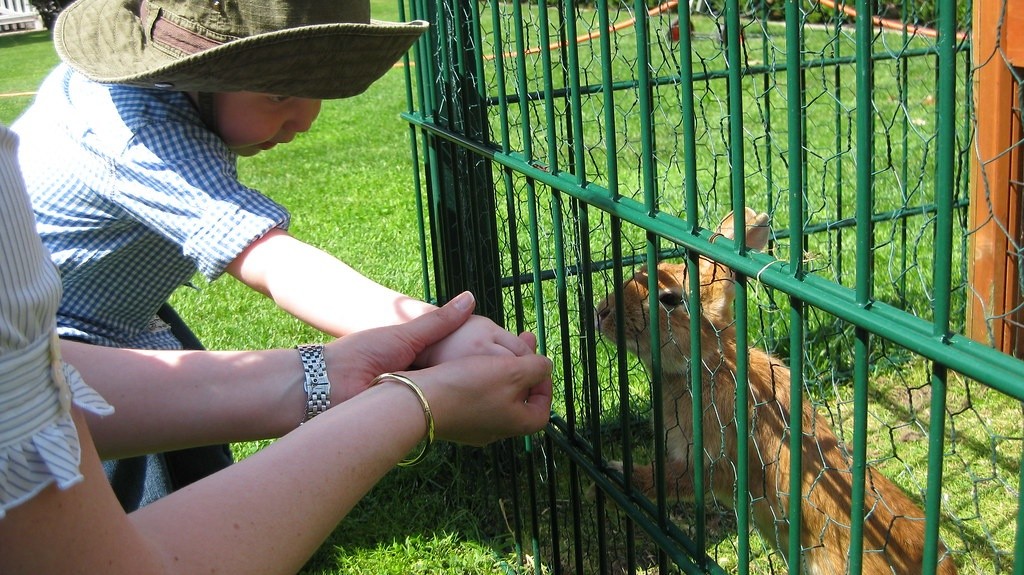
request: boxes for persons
[0,127,555,575]
[0,0,533,513]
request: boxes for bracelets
[294,341,332,427]
[369,373,435,468]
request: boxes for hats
[52,0,430,98]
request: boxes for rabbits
[593,207,960,575]
